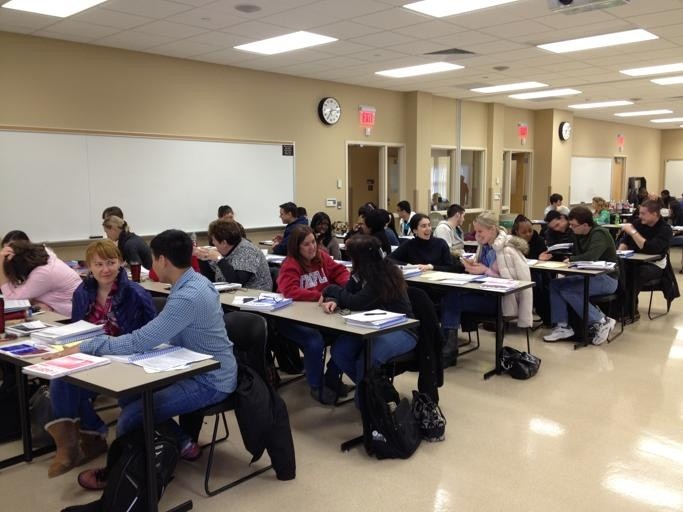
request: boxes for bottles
[0,288,6,334]
[615,212,621,226]
[190,231,197,248]
[609,199,636,210]
[371,430,385,443]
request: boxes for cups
[130,263,141,283]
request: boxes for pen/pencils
[364,312,387,315]
[213,284,224,285]
[459,254,473,264]
[263,296,271,297]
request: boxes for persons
[431,193,449,211]
[543,187,683,345]
[0,207,237,511]
[460,176,469,205]
[509,213,548,259]
[387,204,534,369]
[209,201,418,404]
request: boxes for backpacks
[357,366,401,457]
[104,425,182,512]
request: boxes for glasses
[572,223,583,229]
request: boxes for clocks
[318,97,341,125]
[559,121,572,141]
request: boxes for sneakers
[618,310,641,322]
[76,468,109,490]
[178,438,204,464]
[542,324,575,343]
[591,315,616,345]
[310,386,339,405]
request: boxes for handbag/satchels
[648,252,680,321]
[28,383,54,452]
[369,397,422,461]
[500,346,542,381]
[411,390,447,443]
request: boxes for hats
[358,201,377,215]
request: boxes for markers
[89,235,103,238]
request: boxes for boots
[442,327,460,360]
[324,368,356,397]
[74,428,109,467]
[41,414,86,478]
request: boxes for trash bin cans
[499,213,518,235]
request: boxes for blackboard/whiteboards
[569,156,612,205]
[2,125,296,247]
[664,159,683,198]
